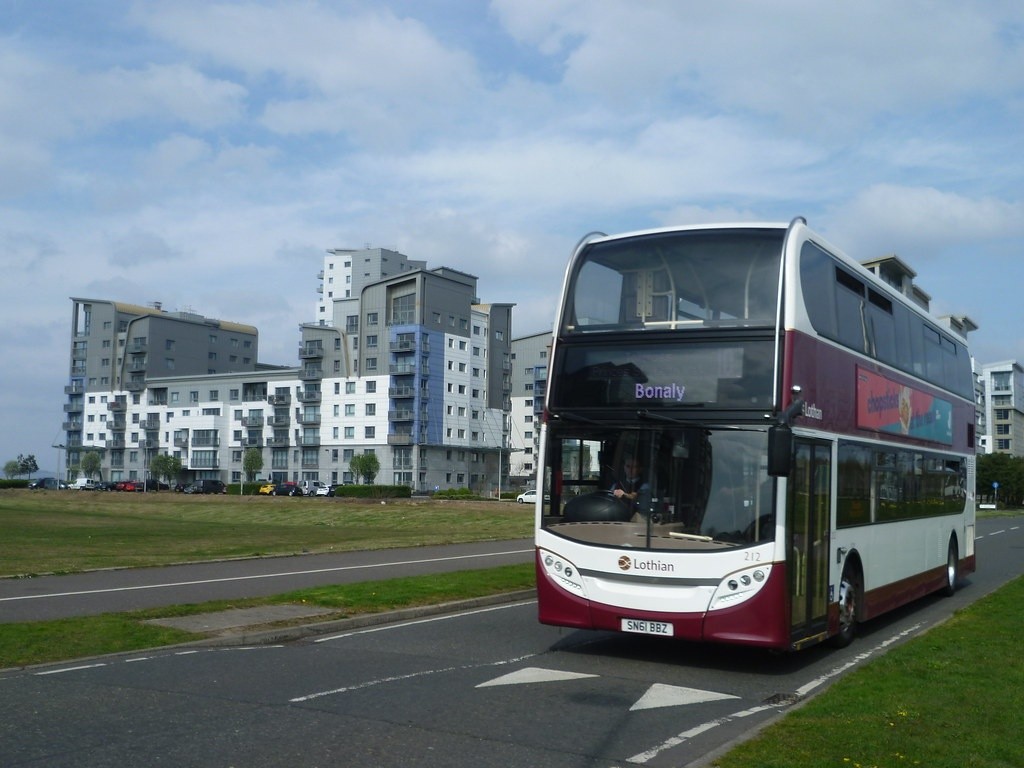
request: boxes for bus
[533,216,979,655]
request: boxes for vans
[300,480,325,497]
[191,478,227,494]
[45,477,70,489]
[28,477,53,490]
[67,478,94,491]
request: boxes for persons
[609,451,651,507]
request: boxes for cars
[329,484,343,496]
[271,484,302,497]
[95,478,170,491]
[175,482,190,492]
[517,490,550,504]
[260,485,277,495]
[316,485,332,496]
